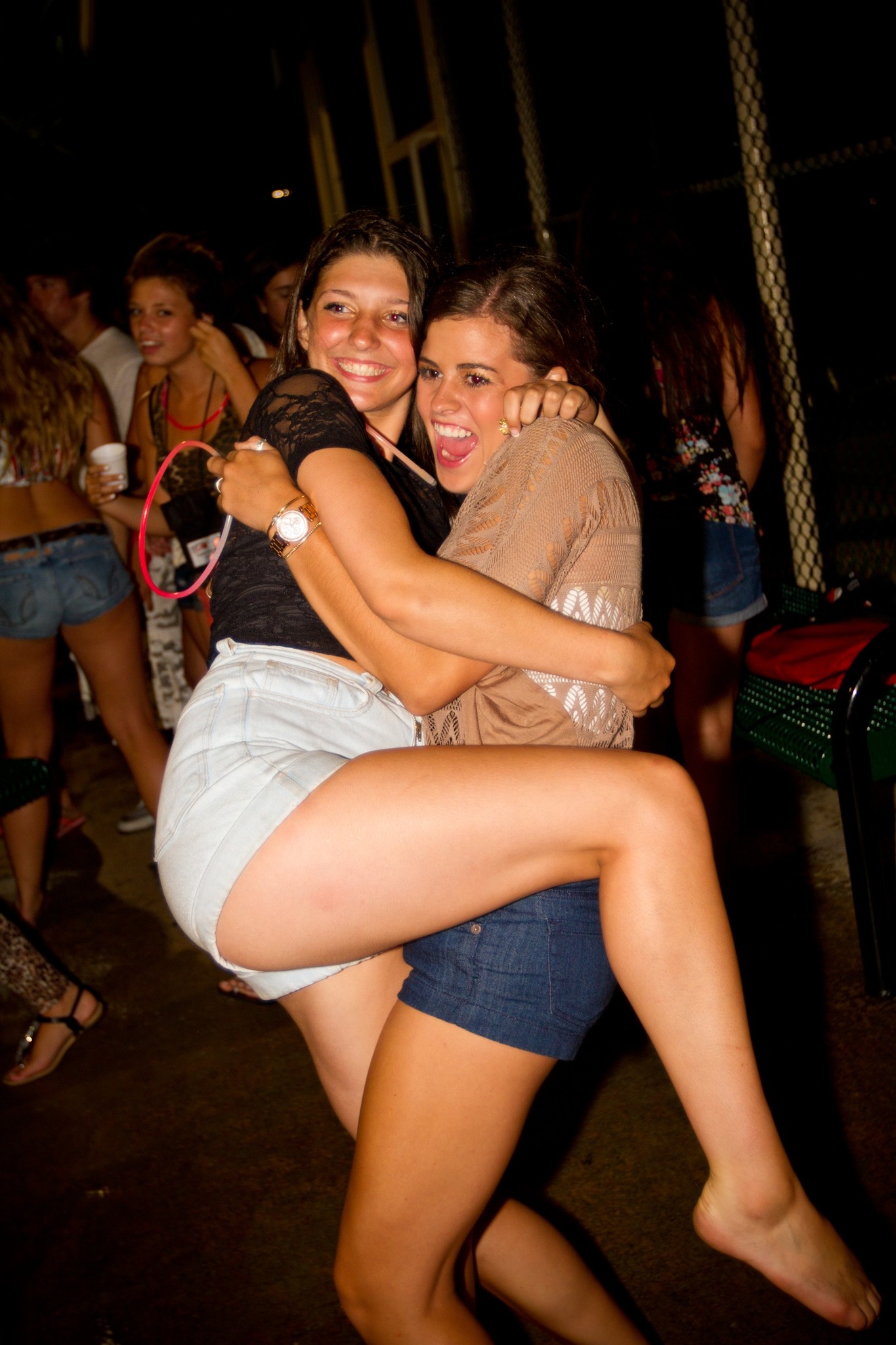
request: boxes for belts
[1,523,110,554]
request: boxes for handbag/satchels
[744,617,896,688]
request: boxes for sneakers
[116,801,155,832]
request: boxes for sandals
[216,974,276,1007]
[3,986,105,1086]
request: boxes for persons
[256,250,308,335]
[573,175,787,874]
[206,263,647,1345]
[0,909,103,1085]
[0,230,276,1002]
[153,212,884,1344]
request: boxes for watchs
[268,500,318,558]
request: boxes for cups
[92,441,131,493]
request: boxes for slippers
[52,814,89,837]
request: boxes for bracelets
[266,493,307,537]
[282,522,321,560]
